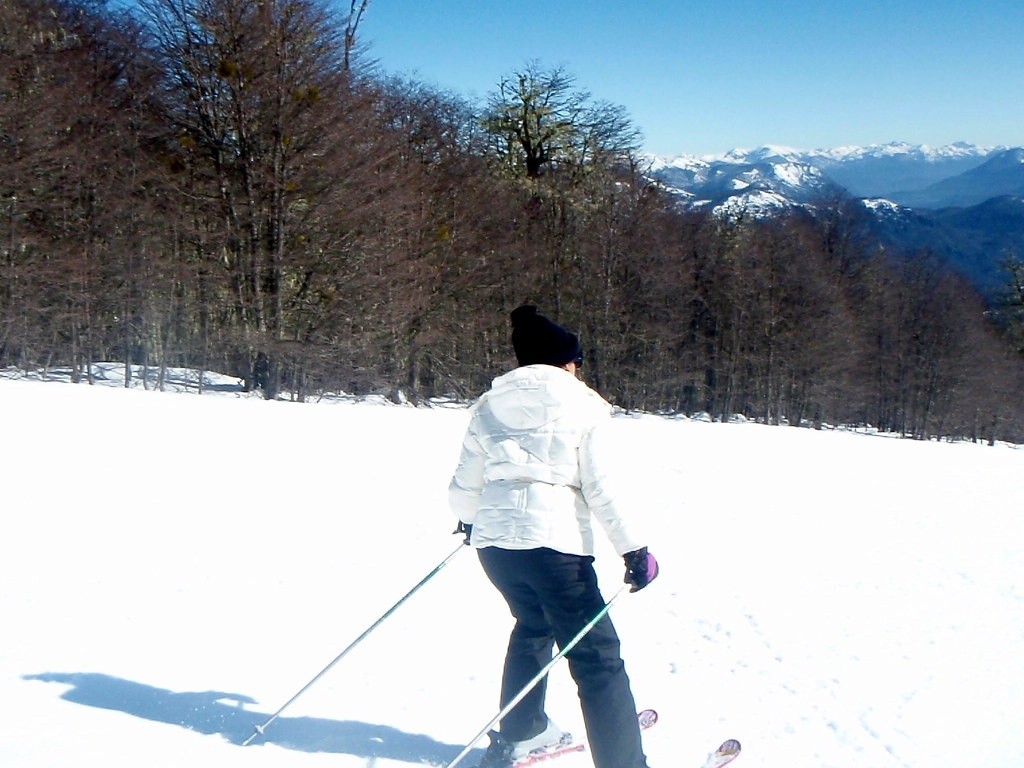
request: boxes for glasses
[572,356,583,367]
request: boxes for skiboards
[478,703,743,768]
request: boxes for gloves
[623,546,659,593]
[462,524,472,545]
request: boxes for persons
[449,305,659,768]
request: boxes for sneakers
[508,719,563,763]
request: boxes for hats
[510,305,579,369]
